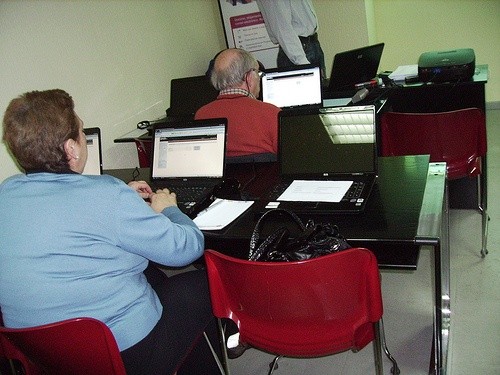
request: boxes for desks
[114,63,488,209]
[102,154,450,375]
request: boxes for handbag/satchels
[248,208,352,262]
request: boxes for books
[193,200,254,230]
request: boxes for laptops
[142,117,229,218]
[257,64,324,111]
[323,42,384,92]
[169,75,220,117]
[255,104,378,217]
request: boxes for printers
[417,47,475,83]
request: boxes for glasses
[258,71,264,78]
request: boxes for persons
[0,88,250,375]
[195,48,280,157]
[257,1,326,79]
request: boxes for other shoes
[226,335,252,359]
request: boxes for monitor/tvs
[81,127,103,176]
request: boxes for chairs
[134,137,154,168]
[0,317,225,375]
[380,108,489,257]
[203,248,400,375]
